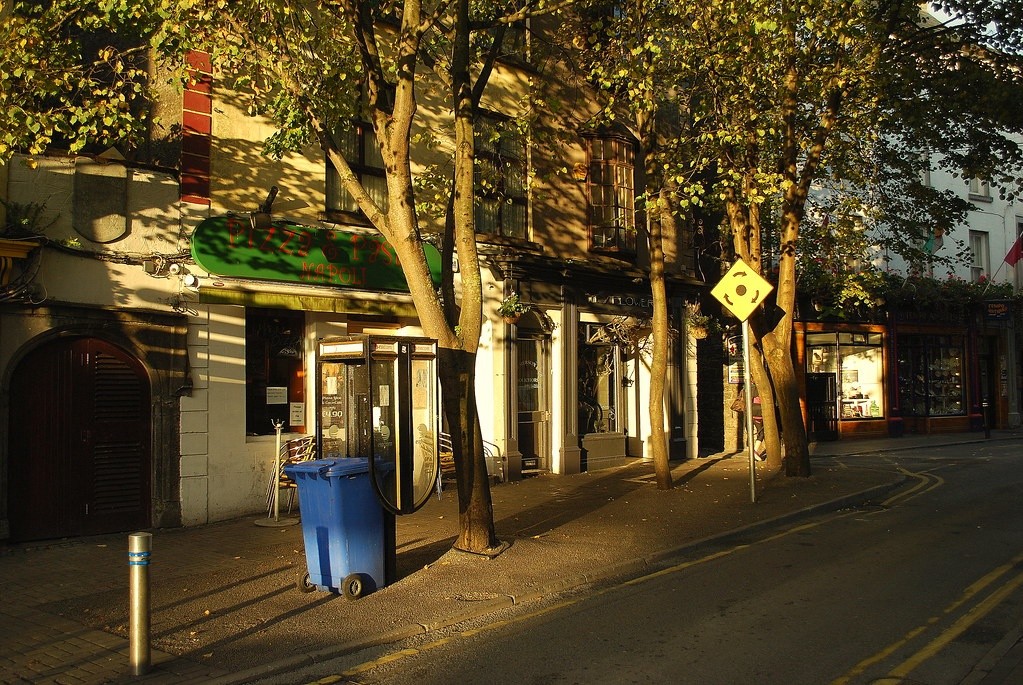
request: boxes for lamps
[226,185,279,229]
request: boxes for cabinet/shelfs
[914,368,960,399]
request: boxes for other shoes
[754,451,762,461]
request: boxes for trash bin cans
[283,456,396,601]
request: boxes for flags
[1004,237,1023,267]
[923,225,944,255]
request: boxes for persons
[744,372,766,461]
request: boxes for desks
[843,399,875,416]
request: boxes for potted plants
[497,292,532,325]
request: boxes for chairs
[265,435,317,520]
[415,430,456,501]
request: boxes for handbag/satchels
[730,390,745,411]
